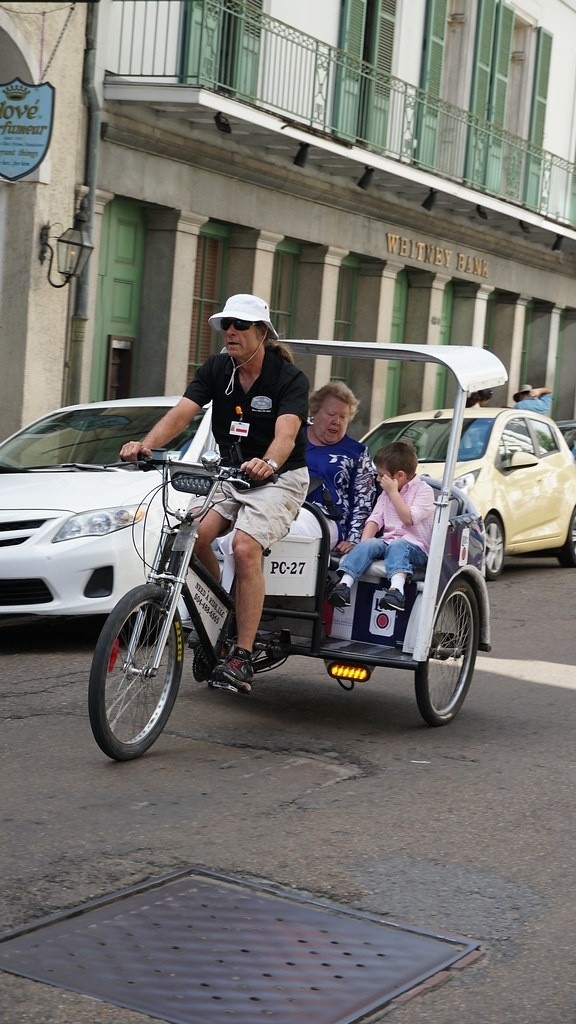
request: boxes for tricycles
[82,338,510,762]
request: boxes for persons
[329,443,437,611]
[513,384,553,414]
[465,388,492,407]
[119,295,310,687]
[187,374,375,646]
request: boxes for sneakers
[188,628,202,650]
[212,654,254,692]
[330,583,351,607]
[378,588,405,611]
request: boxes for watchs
[263,458,279,473]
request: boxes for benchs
[328,478,462,642]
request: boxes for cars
[351,404,576,581]
[0,394,218,631]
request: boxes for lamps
[211,111,233,134]
[356,168,373,191]
[511,50,525,62]
[519,223,531,234]
[551,235,564,251]
[420,190,440,212]
[451,12,465,29]
[291,142,310,168]
[34,179,94,301]
[473,206,488,220]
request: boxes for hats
[513,384,532,403]
[207,293,280,340]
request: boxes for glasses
[220,318,262,332]
